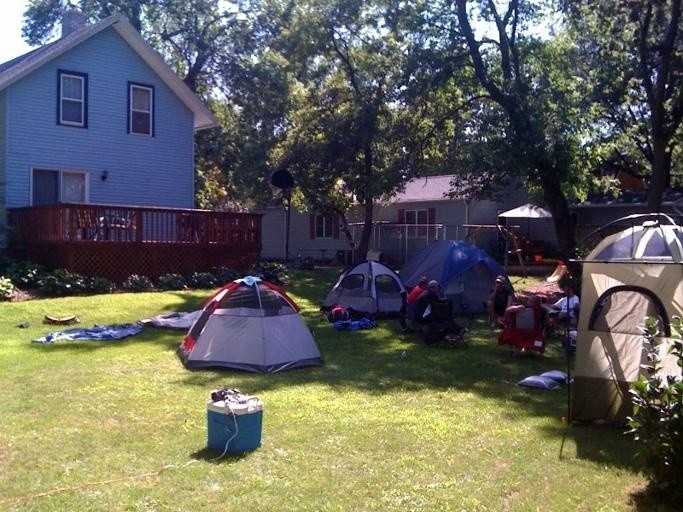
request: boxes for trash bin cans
[335,249,352,265]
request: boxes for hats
[426,280,444,289]
[496,274,506,282]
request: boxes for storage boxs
[207,400,263,452]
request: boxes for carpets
[29,323,144,340]
[135,309,201,329]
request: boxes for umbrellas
[498,202,552,239]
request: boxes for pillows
[540,369,569,379]
[518,375,560,390]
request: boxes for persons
[548,283,580,324]
[486,275,513,327]
[406,275,472,346]
[515,235,542,261]
[497,294,554,332]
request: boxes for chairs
[399,289,475,350]
[486,287,579,360]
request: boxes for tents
[177,275,325,377]
[399,237,515,316]
[320,259,408,321]
[574,210,683,425]
